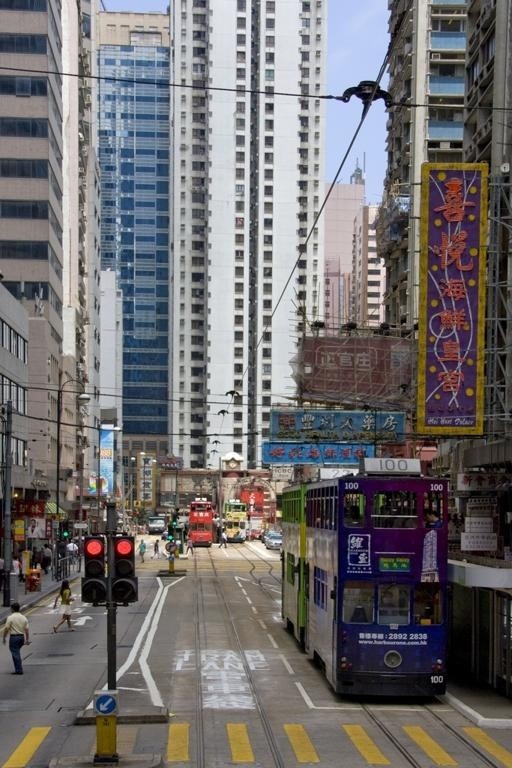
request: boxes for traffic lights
[84,537,136,603]
[167,513,177,540]
[60,520,73,538]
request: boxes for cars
[246,528,282,550]
[149,520,166,535]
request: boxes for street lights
[97,426,122,520]
[55,380,90,543]
[128,451,157,510]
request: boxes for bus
[188,498,246,547]
[280,458,447,696]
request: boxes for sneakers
[10,672,24,675]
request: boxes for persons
[151,539,162,559]
[185,535,194,555]
[53,580,77,633]
[423,508,444,528]
[219,528,228,548]
[136,540,146,563]
[344,505,365,528]
[0,522,131,592]
[162,529,168,541]
[3,603,32,675]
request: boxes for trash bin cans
[26,569,42,592]
